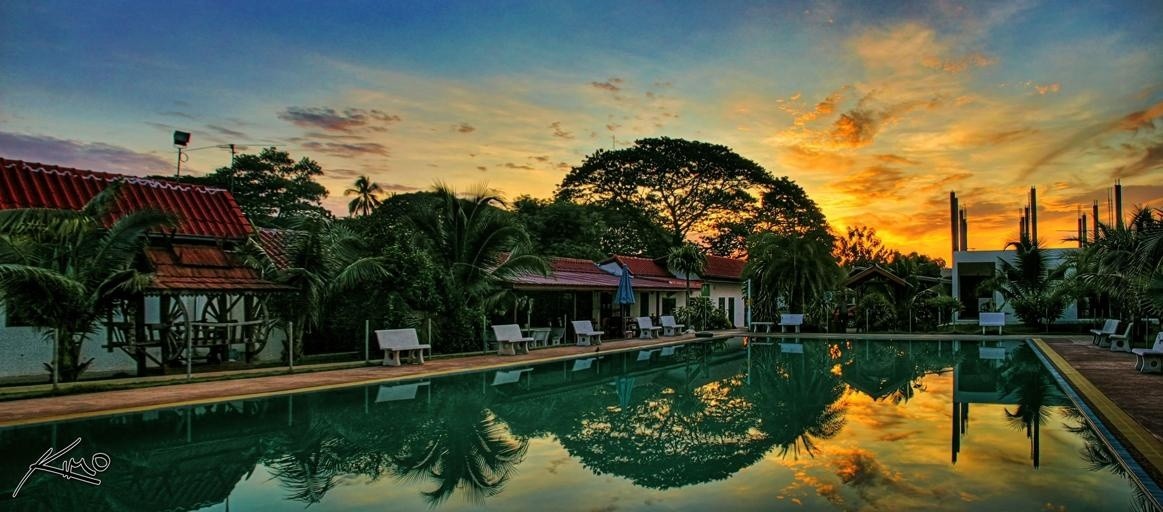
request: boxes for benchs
[977,312,1005,334]
[372,328,434,369]
[569,319,604,346]
[751,314,804,334]
[635,315,686,339]
[490,322,531,356]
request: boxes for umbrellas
[612,351,636,416]
[611,263,636,340]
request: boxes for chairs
[1089,318,1135,353]
[1130,331,1162,374]
